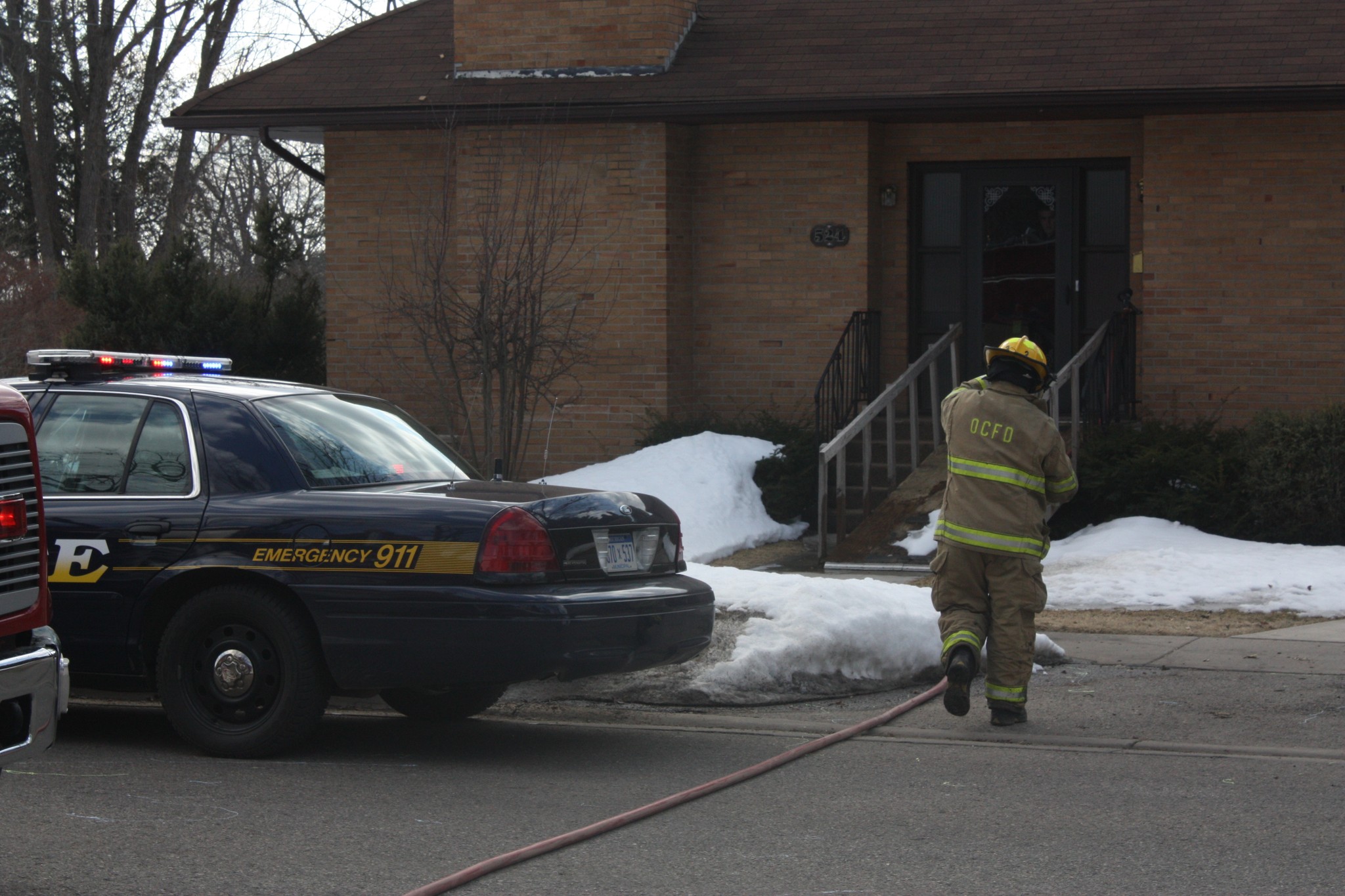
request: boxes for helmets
[982,335,1049,394]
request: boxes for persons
[929,335,1080,726]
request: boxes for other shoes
[989,707,1027,725]
[943,649,975,717]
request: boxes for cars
[0,347,718,759]
[0,381,71,767]
